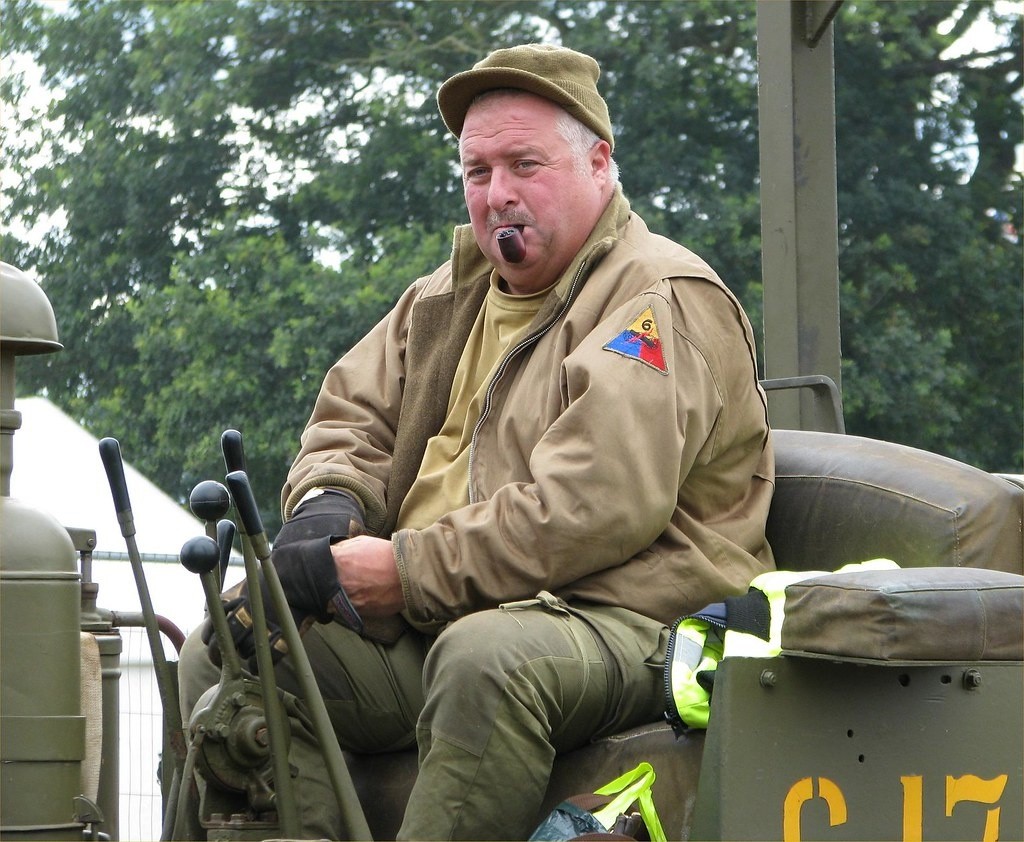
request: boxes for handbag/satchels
[525,762,667,842]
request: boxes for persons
[177,44,774,842]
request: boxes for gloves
[200,534,364,676]
[270,489,367,552]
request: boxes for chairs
[343,430,1024,842]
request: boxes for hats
[437,44,615,156]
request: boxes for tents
[11,396,262,842]
